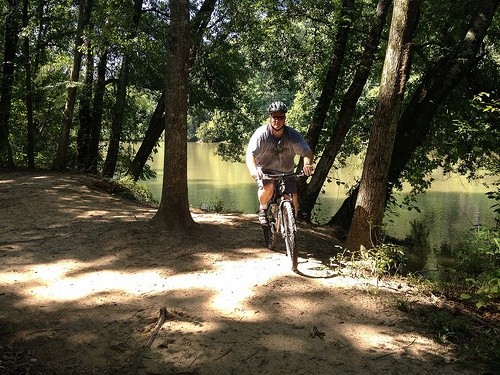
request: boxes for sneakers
[259,216,269,226]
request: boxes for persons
[246,101,314,233]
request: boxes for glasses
[271,116,286,120]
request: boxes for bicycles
[257,168,315,272]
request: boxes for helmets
[268,101,287,113]
